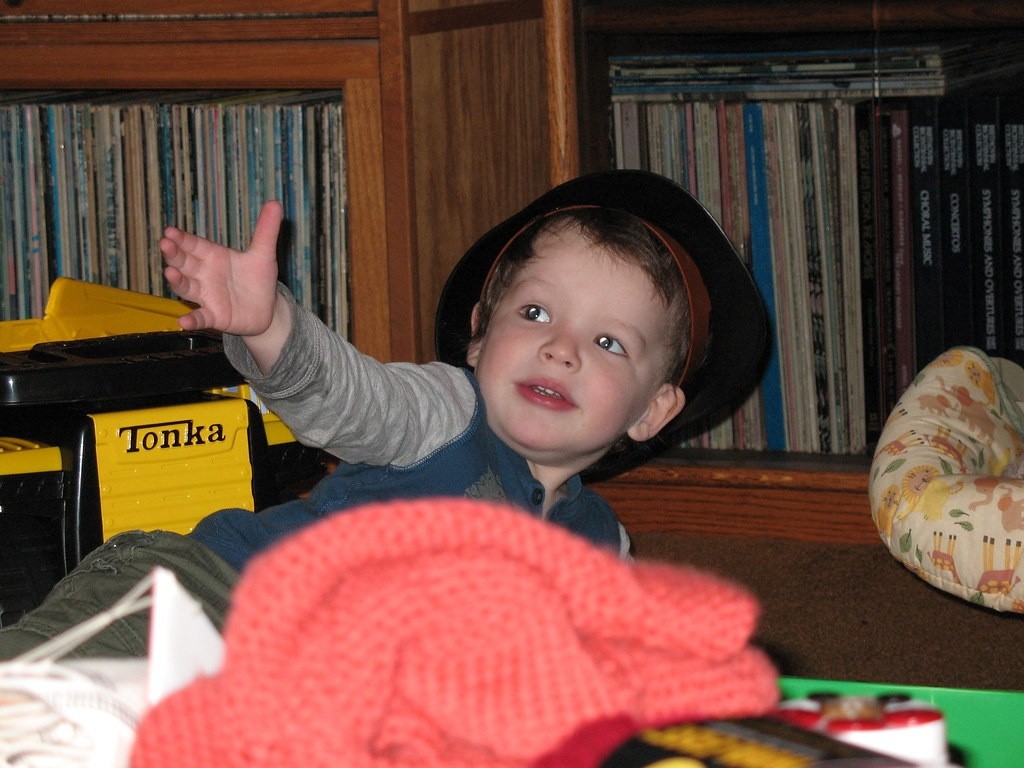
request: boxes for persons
[0,167,774,676]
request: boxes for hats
[432,169,776,488]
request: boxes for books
[0,87,349,355]
[608,31,1024,455]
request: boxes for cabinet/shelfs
[0,0,1024,548]
